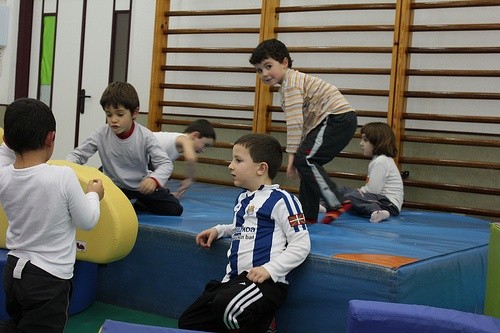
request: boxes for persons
[0,98,105,333]
[249,39,357,225]
[66,81,217,216]
[177,134,310,333]
[318,122,404,223]
